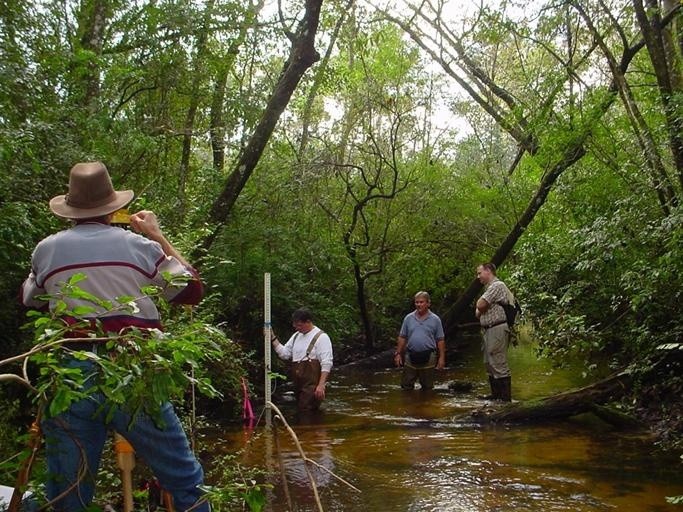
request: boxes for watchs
[393,351,401,357]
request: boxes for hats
[49,160,135,220]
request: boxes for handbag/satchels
[407,348,433,368]
[497,299,523,327]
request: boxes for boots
[477,376,512,403]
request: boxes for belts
[481,320,508,331]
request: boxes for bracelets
[271,336,277,343]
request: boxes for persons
[392,290,445,391]
[17,160,218,512]
[473,260,521,405]
[261,306,334,414]
[264,431,335,489]
